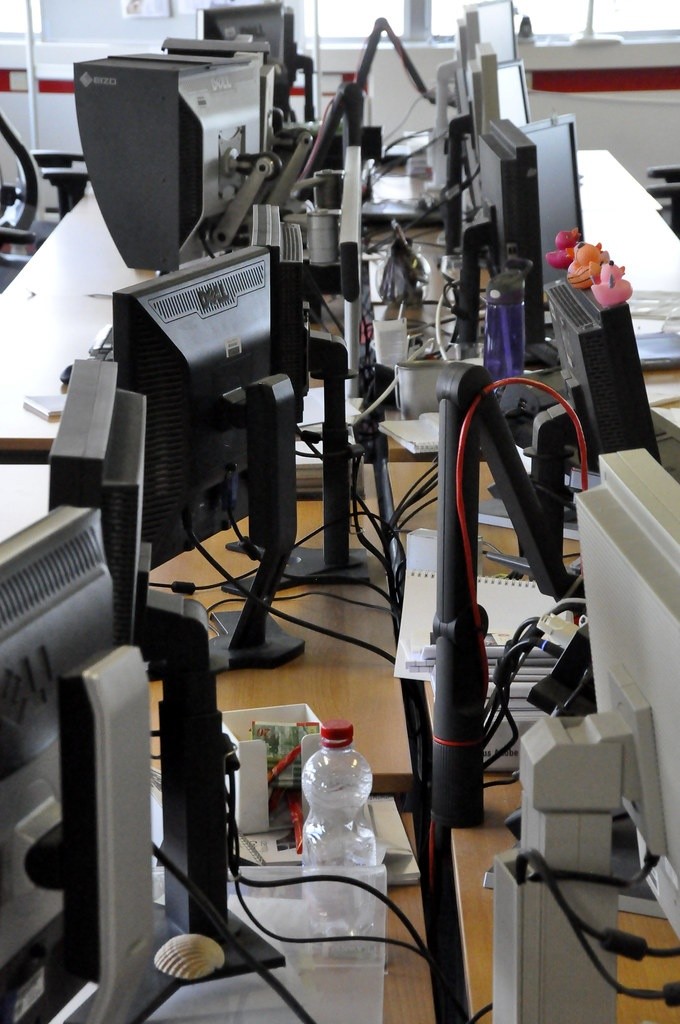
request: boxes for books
[393,569,579,720]
[295,386,363,494]
[478,499,579,540]
[378,412,439,454]
[229,796,421,885]
[24,394,68,416]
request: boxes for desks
[0,148,680,1024]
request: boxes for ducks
[546,227,632,306]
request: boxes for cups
[394,359,447,420]
[441,255,462,302]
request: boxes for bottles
[301,720,376,960]
[484,260,526,382]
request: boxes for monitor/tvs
[0,0,680,1024]
